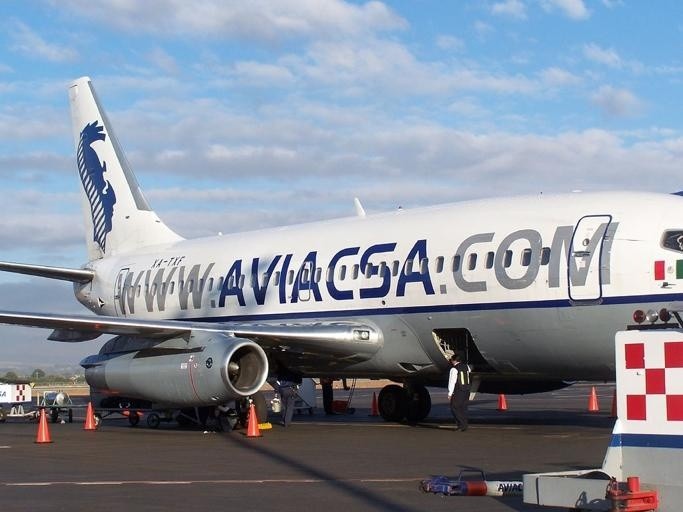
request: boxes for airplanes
[0,69,682,434]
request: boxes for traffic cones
[605,386,616,419]
[367,392,382,419]
[32,408,53,444]
[244,403,265,439]
[498,393,508,410]
[588,384,599,412]
[82,400,99,433]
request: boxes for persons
[448,353,472,434]
[342,377,350,390]
[274,370,302,428]
[320,377,337,414]
